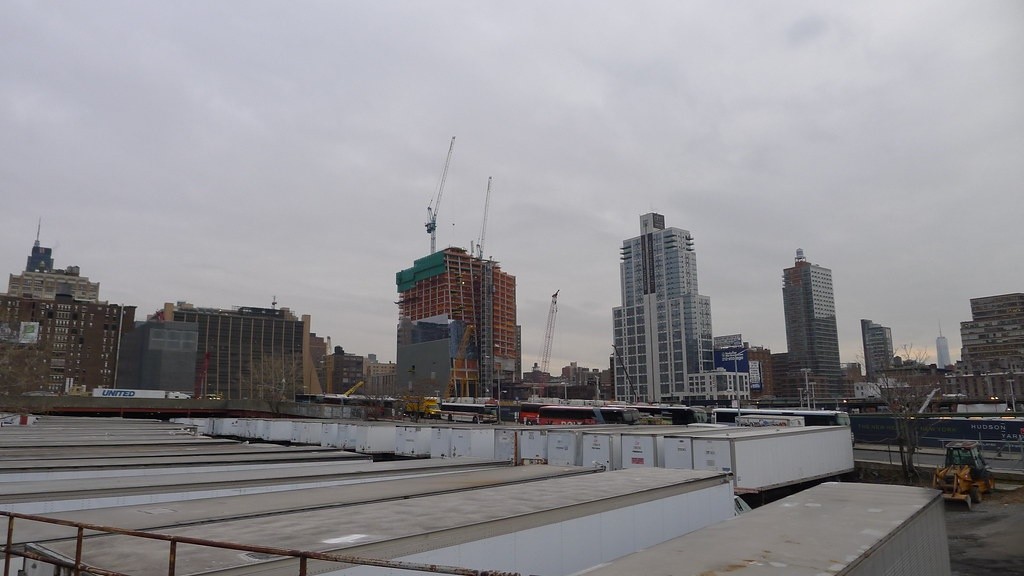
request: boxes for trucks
[0,418,955,576]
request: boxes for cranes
[611,345,640,401]
[477,177,492,260]
[537,290,562,398]
[426,136,456,256]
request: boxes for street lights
[798,368,816,411]
[1006,379,1016,412]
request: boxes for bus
[295,394,851,426]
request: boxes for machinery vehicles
[933,441,995,511]
